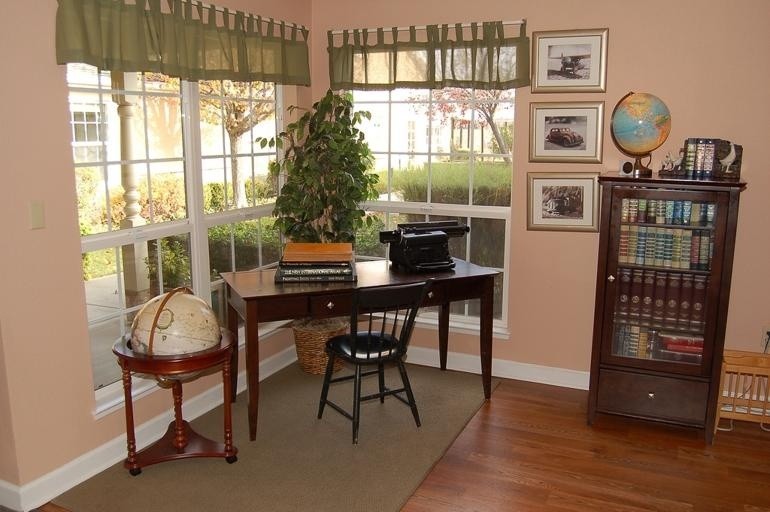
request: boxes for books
[273,242,358,283]
[611,198,717,366]
[685,137,743,180]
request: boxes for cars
[546,126,585,148]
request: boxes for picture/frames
[531,27,609,93]
[526,171,599,233]
[528,101,605,165]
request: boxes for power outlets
[762,327,770,352]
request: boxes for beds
[713,350,770,434]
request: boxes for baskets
[292,321,348,375]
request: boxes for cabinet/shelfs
[112,326,238,476]
[586,176,746,444]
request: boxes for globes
[610,91,671,175]
[111,286,238,476]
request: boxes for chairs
[317,277,435,447]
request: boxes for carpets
[50,359,503,512]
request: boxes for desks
[218,256,503,442]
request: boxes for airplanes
[548,52,591,74]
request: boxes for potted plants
[255,88,381,375]
